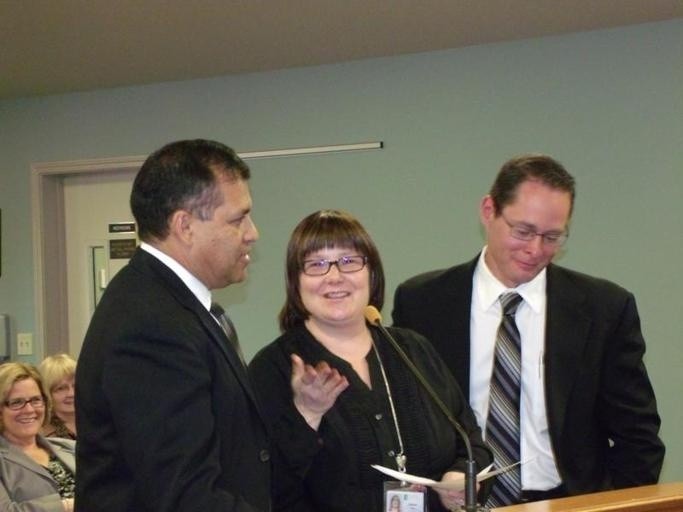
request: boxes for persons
[36,351,76,439]
[0,360,74,512]
[248,208,495,511]
[388,494,400,512]
[71,137,275,512]
[390,155,665,511]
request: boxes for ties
[210,302,248,377]
[482,291,524,510]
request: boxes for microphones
[363,305,481,512]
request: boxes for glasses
[300,253,368,277]
[4,395,46,411]
[498,210,570,248]
